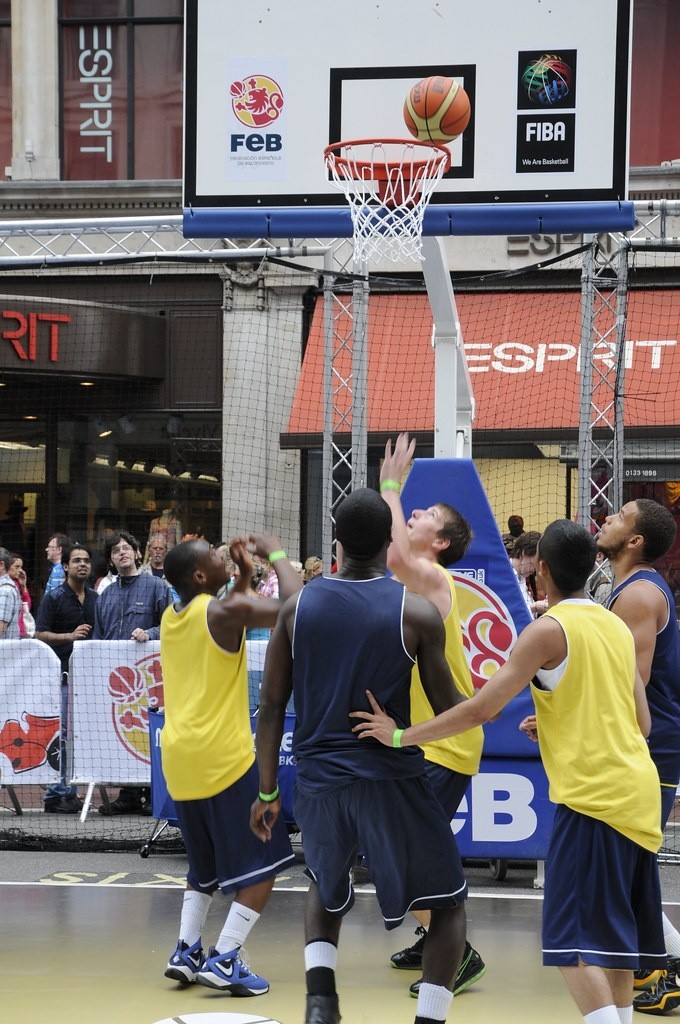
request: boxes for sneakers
[390,927,426,970]
[409,941,487,998]
[164,939,202,984]
[44,795,92,815]
[97,791,143,815]
[633,956,680,1013]
[197,946,269,997]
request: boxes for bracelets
[269,550,287,564]
[259,785,279,802]
[392,729,403,748]
[380,481,400,492]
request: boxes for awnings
[279,290,680,434]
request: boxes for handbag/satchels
[21,601,35,638]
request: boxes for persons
[378,431,485,998]
[0,532,338,813]
[500,514,615,620]
[143,509,182,565]
[250,489,503,1024]
[349,520,663,1024]
[160,534,302,997]
[593,499,680,1012]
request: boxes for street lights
[560,455,578,519]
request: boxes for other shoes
[305,994,341,1024]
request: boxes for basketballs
[402,75,471,147]
